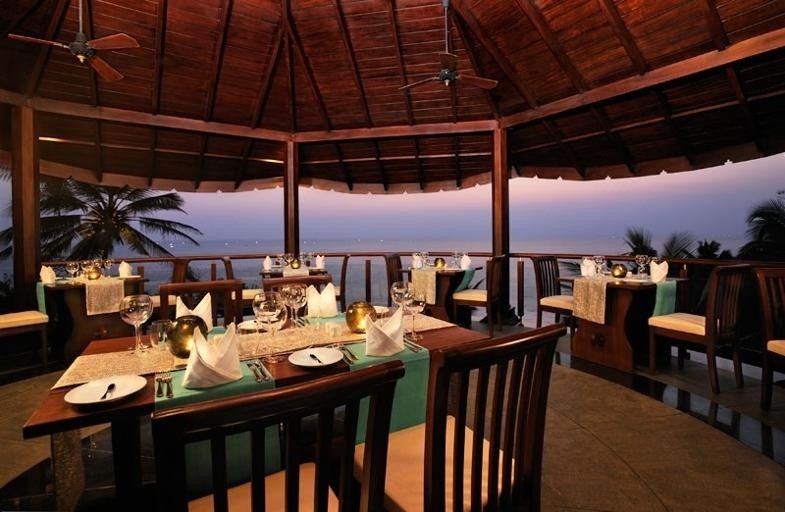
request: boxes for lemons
[611,265,627,278]
[345,301,377,335]
[435,258,445,268]
[159,315,208,359]
[84,267,101,279]
[289,259,301,269]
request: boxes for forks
[161,370,174,398]
[155,371,163,397]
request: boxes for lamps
[355,322,568,511]
[756,268,785,412]
[159,279,245,330]
[0,310,50,370]
[452,253,509,337]
[150,359,407,512]
[220,255,265,327]
[263,274,332,318]
[149,257,191,323]
[334,253,352,314]
[529,255,579,353]
[647,262,753,394]
[383,252,410,307]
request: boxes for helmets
[288,347,343,369]
[119,276,140,278]
[64,373,147,407]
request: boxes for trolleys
[7,1,141,85]
[396,1,499,92]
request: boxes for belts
[149,320,173,368]
[257,302,287,336]
[595,255,606,276]
[285,254,294,267]
[119,295,152,353]
[81,259,94,281]
[278,284,308,322]
[92,257,102,269]
[105,259,114,278]
[252,292,284,364]
[637,254,647,277]
[452,250,460,266]
[65,262,79,284]
[391,282,414,309]
[278,284,295,322]
[582,255,593,276]
[403,287,426,342]
